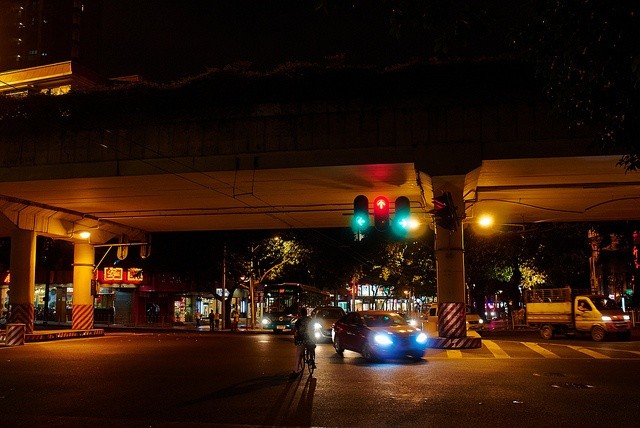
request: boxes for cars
[333,310,428,360]
[310,307,346,342]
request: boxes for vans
[429,302,483,335]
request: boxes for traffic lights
[394,196,411,236]
[353,194,369,235]
[374,196,390,233]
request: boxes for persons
[148,304,160,324]
[209,310,214,331]
[578,302,588,310]
[194,309,203,327]
[291,307,316,374]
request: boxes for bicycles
[293,331,316,375]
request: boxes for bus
[263,285,329,334]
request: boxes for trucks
[526,288,633,341]
